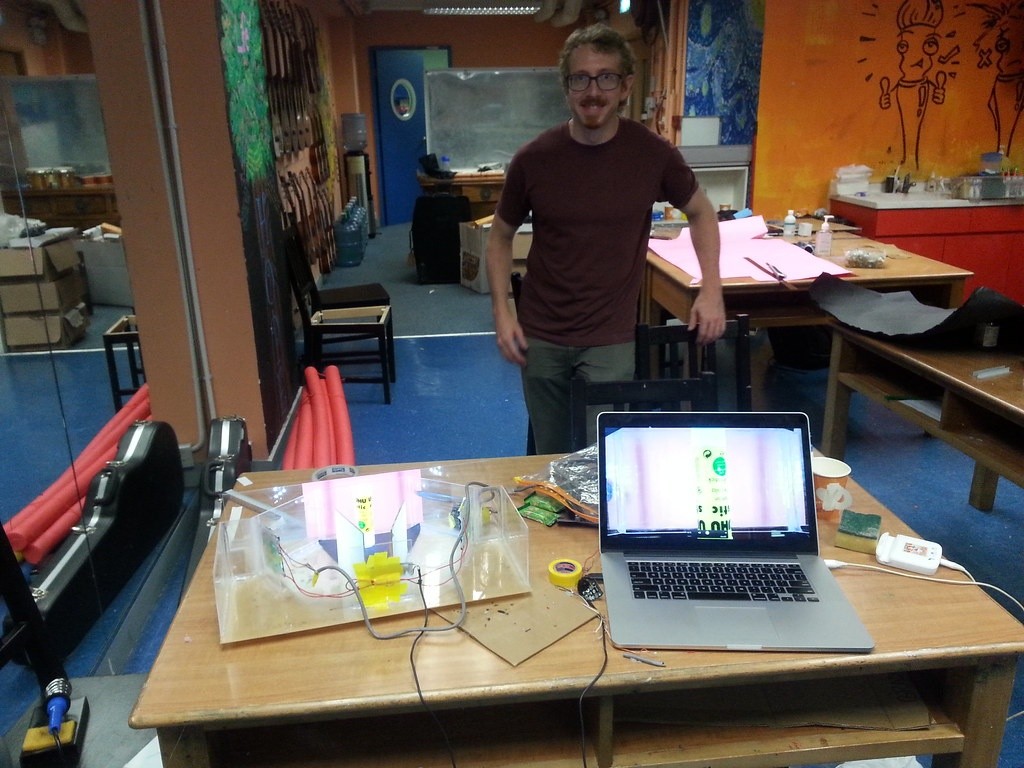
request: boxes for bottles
[926,144,1024,199]
[341,113,368,153]
[440,155,450,173]
[974,300,1001,352]
[783,209,797,239]
[333,195,369,267]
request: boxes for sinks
[840,191,970,208]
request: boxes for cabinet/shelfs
[830,199,1024,303]
[414,172,507,220]
[0,190,121,234]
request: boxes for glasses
[563,69,626,93]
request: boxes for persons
[484,24,728,455]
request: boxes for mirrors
[0,0,206,748]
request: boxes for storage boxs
[82,237,133,306]
[459,221,534,294]
[0,239,90,353]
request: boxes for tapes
[548,558,582,587]
[311,465,358,481]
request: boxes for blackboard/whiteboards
[6,74,111,176]
[423,67,573,170]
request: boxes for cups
[811,456,852,523]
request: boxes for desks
[128,446,1022,768]
[821,316,1024,490]
[638,232,975,412]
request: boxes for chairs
[510,270,539,456]
[572,315,752,451]
[409,196,472,284]
[281,210,395,404]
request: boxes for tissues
[829,163,875,195]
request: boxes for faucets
[902,171,916,193]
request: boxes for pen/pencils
[416,490,462,503]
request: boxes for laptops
[596,412,875,654]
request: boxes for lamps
[421,0,542,16]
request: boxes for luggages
[411,194,472,284]
